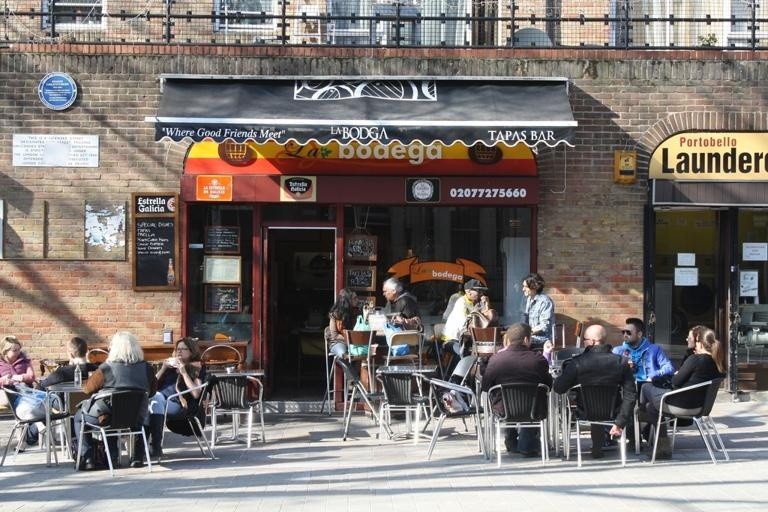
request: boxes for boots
[129,424,149,468]
[639,435,673,462]
[636,404,648,424]
[149,412,167,459]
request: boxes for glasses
[621,329,633,336]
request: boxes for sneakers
[79,449,100,470]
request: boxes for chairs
[642,374,728,466]
[0,340,271,476]
[320,322,655,470]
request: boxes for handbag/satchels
[441,389,471,414]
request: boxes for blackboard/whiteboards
[357,296,376,311]
[203,255,241,284]
[204,225,241,254]
[131,192,179,291]
[204,284,241,313]
[345,265,377,291]
[345,234,378,261]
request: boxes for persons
[0,335,68,446]
[13,335,103,454]
[130,337,206,469]
[73,331,158,470]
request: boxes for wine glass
[46,358,57,374]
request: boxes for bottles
[72,363,83,387]
[166,258,176,286]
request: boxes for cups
[226,366,236,374]
[168,357,180,368]
[551,352,559,363]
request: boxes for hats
[463,279,490,292]
